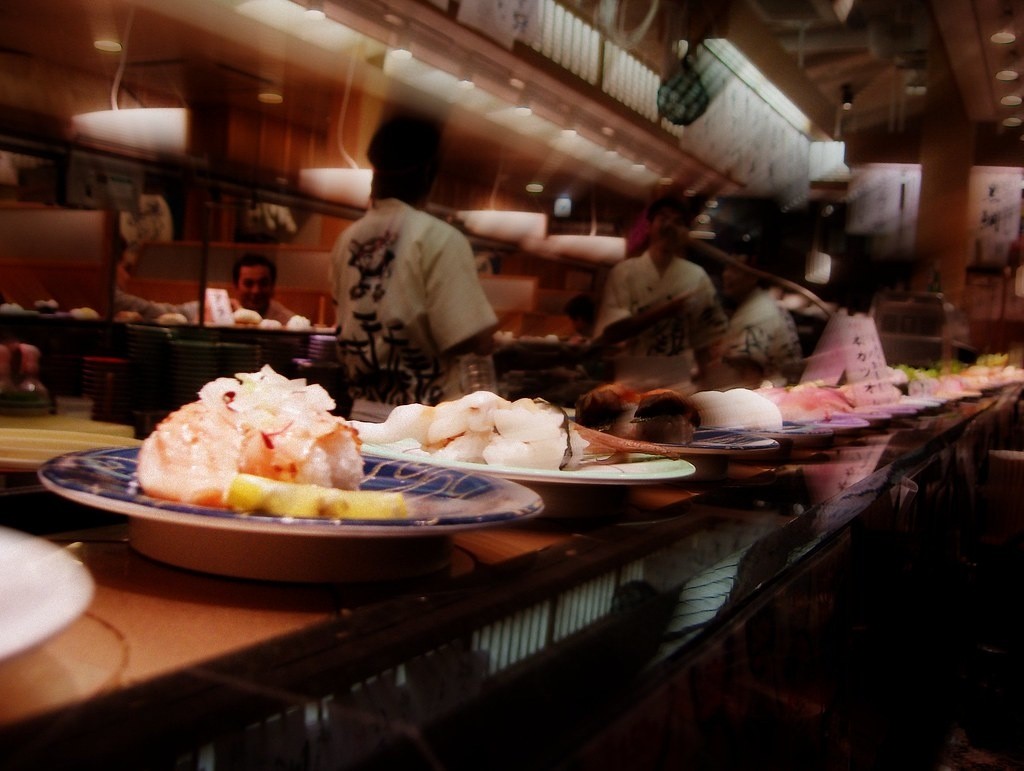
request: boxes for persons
[713,243,803,390]
[729,356,773,393]
[590,189,732,399]
[114,247,299,329]
[564,291,603,337]
[326,102,500,425]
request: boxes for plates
[702,372,1024,452]
[0,523,95,667]
[351,433,700,511]
[565,415,781,479]
[37,443,545,584]
